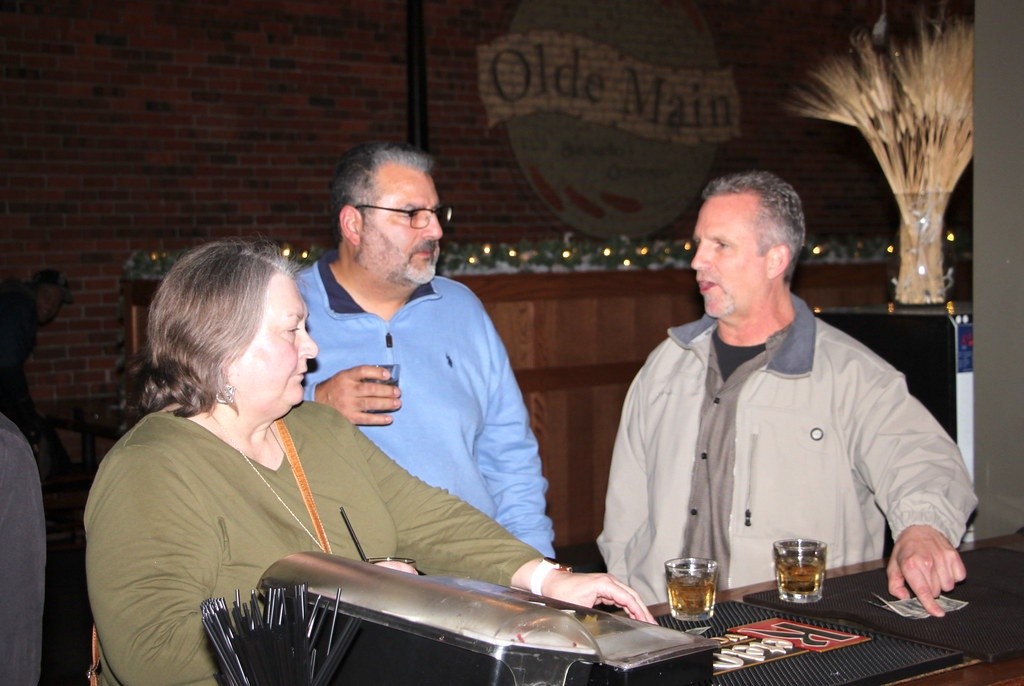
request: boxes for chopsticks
[200,580,361,686]
[338,505,366,562]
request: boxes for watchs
[531,557,573,596]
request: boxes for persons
[0,412,46,686]
[84,241,660,686]
[293,138,575,571]
[0,271,74,462]
[596,171,978,617]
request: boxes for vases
[896,191,950,305]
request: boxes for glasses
[355,203,455,229]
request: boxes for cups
[664,557,719,622]
[361,558,416,570]
[773,539,828,603]
[360,364,399,413]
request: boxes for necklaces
[213,416,334,554]
